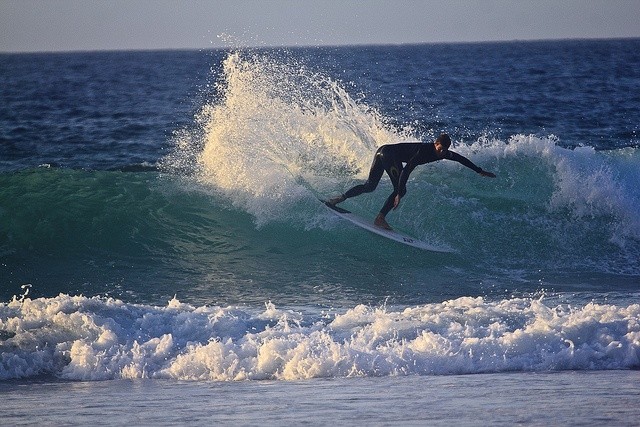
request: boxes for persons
[329,134,496,230]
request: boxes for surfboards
[285,164,459,256]
